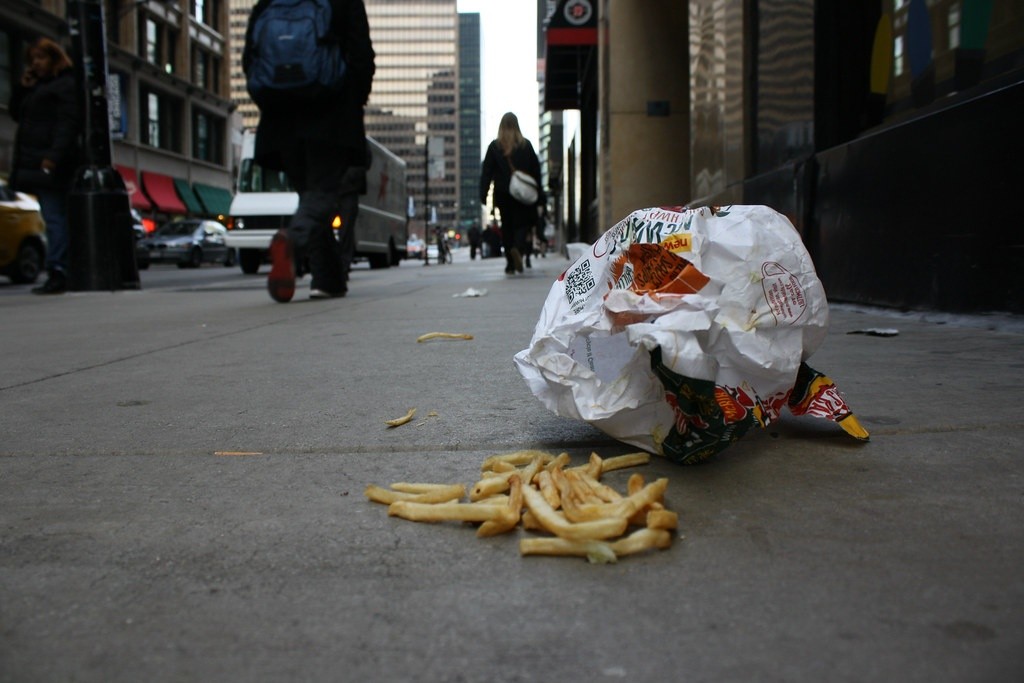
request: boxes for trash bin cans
[481,239,491,258]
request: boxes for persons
[8,36,91,295]
[478,113,544,272]
[465,216,550,269]
[246,0,376,306]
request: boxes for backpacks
[246,0,346,113]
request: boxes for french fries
[364,449,679,560]
[417,333,475,340]
[384,408,416,426]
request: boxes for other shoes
[43,271,83,294]
[505,262,517,274]
[511,247,526,273]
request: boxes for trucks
[222,135,407,274]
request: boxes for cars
[134,218,239,271]
[0,178,48,286]
[406,233,426,260]
[129,209,150,270]
[427,245,439,260]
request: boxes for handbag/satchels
[508,170,538,203]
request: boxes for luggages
[67,160,140,287]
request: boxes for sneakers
[267,229,296,302]
[309,285,339,298]
